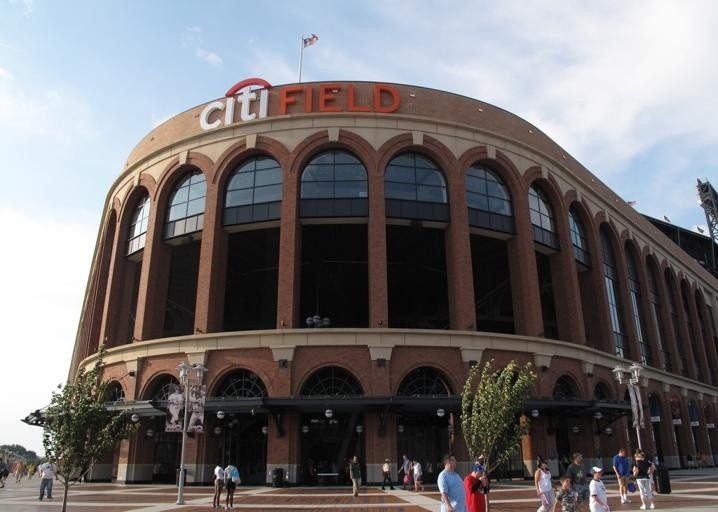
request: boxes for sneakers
[650,503,655,510]
[620,497,633,504]
[639,504,647,510]
[212,503,237,511]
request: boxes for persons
[588,466,611,512]
[612,447,660,510]
[436,454,466,512]
[304,455,362,496]
[536,455,541,469]
[223,459,239,508]
[212,460,225,508]
[553,476,582,512]
[464,466,489,511]
[687,453,705,472]
[535,461,556,512]
[567,453,588,512]
[0,458,56,501]
[381,454,424,491]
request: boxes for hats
[590,466,604,476]
[573,453,583,458]
[471,464,483,474]
[539,456,551,464]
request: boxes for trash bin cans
[272,468,284,487]
[653,466,671,494]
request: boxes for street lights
[174,361,209,507]
[612,362,646,452]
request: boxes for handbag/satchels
[404,474,414,485]
[231,471,242,485]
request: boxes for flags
[302,33,318,47]
